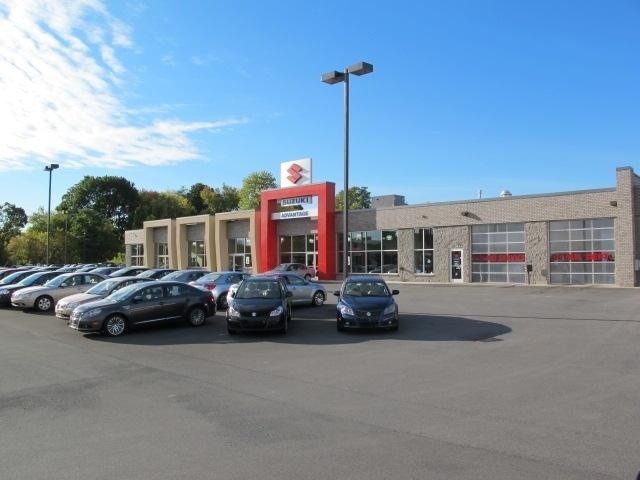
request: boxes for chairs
[347,284,386,296]
[264,282,280,297]
[151,289,162,298]
[144,289,153,299]
[244,283,262,297]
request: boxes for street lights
[42,163,60,264]
[321,63,374,279]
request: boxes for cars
[264,263,316,282]
[228,273,328,308]
[347,263,398,273]
[0,262,254,339]
[226,276,293,336]
[332,274,402,334]
